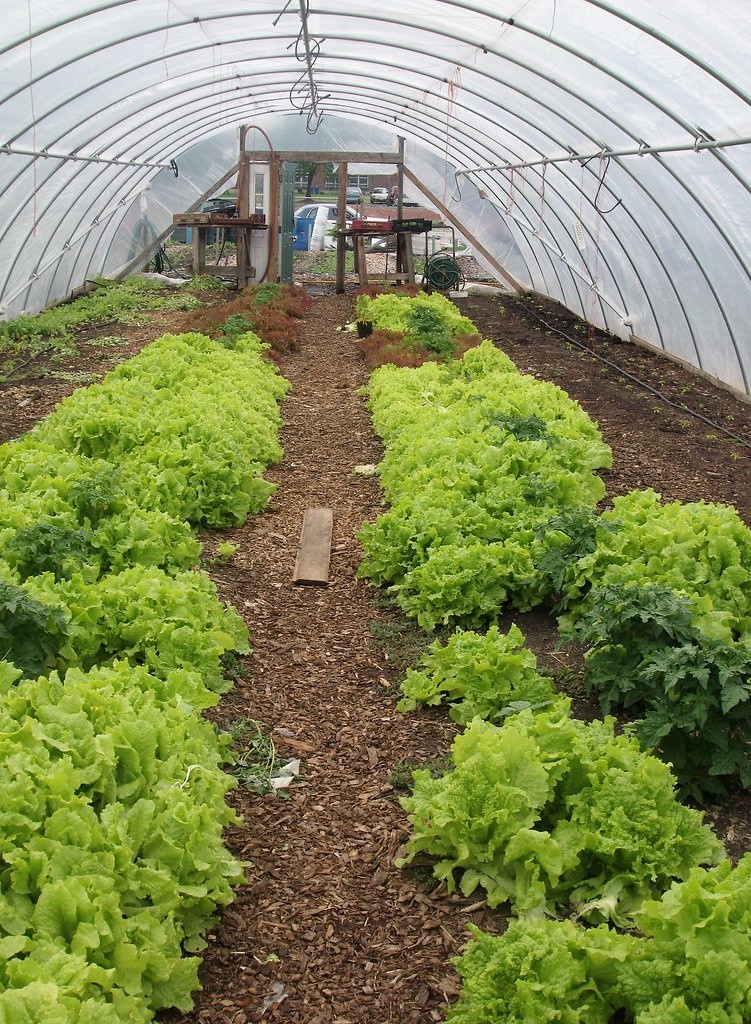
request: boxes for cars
[195,198,235,218]
[293,203,390,252]
[346,186,365,205]
[369,187,390,204]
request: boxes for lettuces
[0,277,750,1024]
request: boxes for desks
[177,225,269,290]
[339,231,421,286]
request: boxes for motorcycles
[386,191,396,205]
